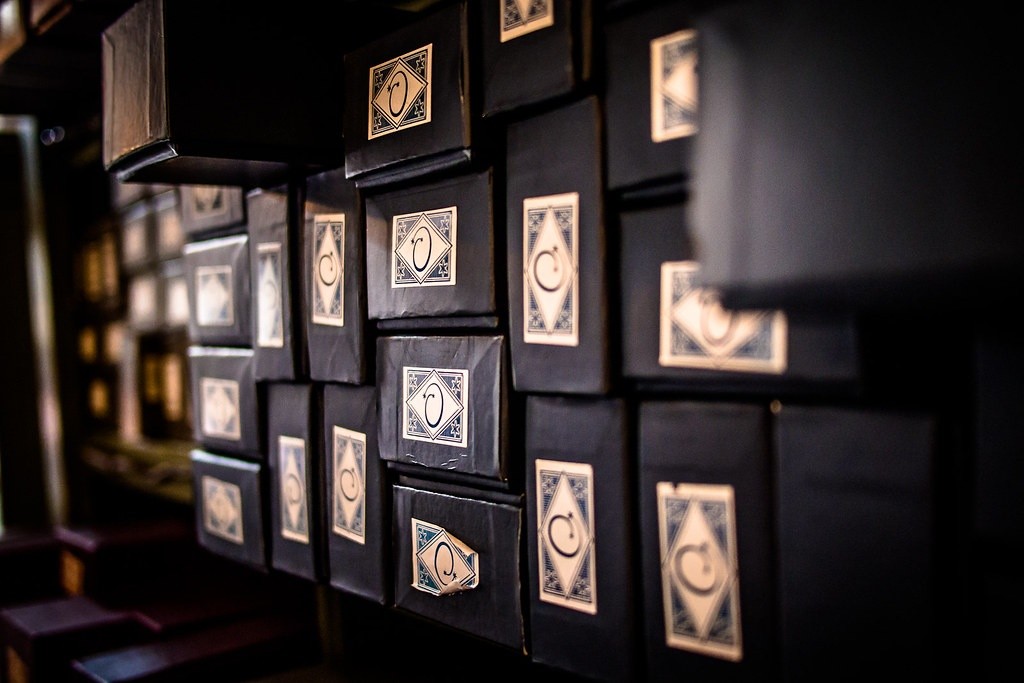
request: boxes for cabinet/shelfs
[0,0,1024,683]
